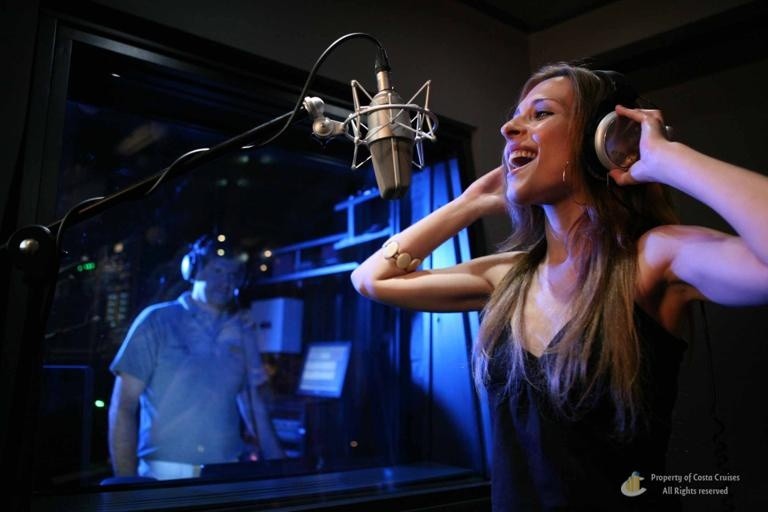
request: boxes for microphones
[366,57,410,200]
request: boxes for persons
[349,62,768,512]
[107,231,286,480]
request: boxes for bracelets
[382,239,421,274]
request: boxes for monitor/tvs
[297,341,353,400]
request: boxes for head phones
[180,233,211,282]
[593,109,676,172]
